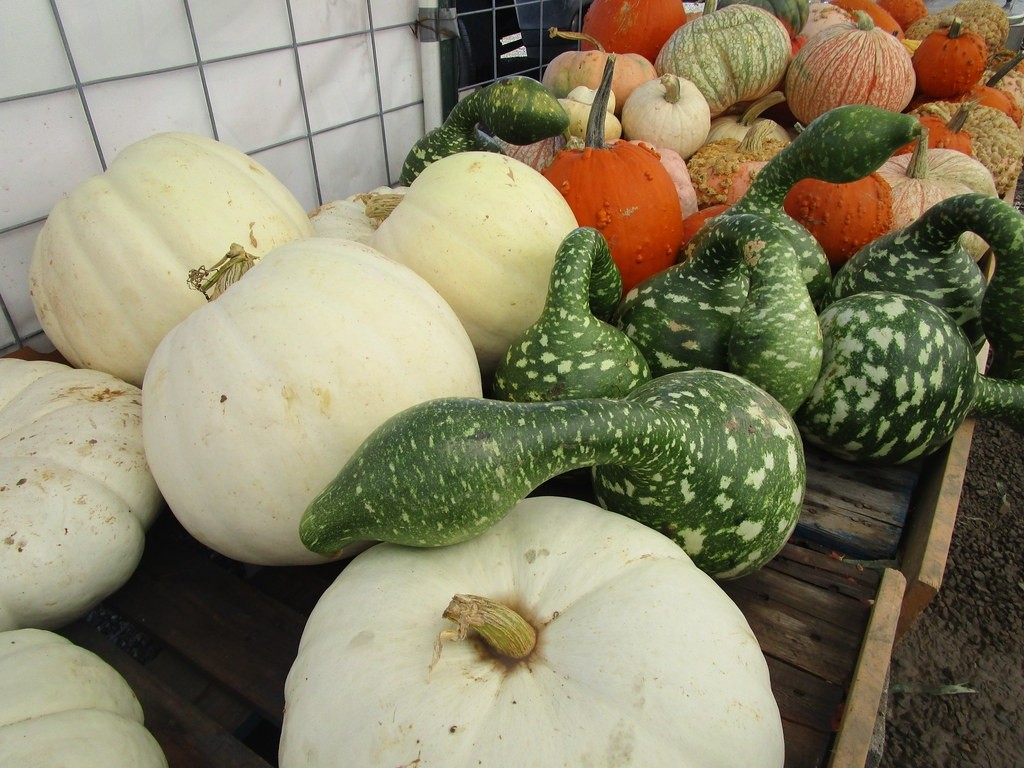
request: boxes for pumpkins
[0,0,1024,768]
